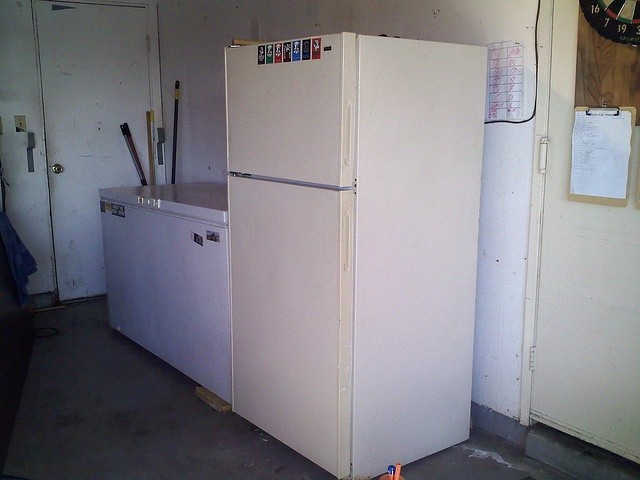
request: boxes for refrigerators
[224,32,489,480]
[98,182,232,407]
